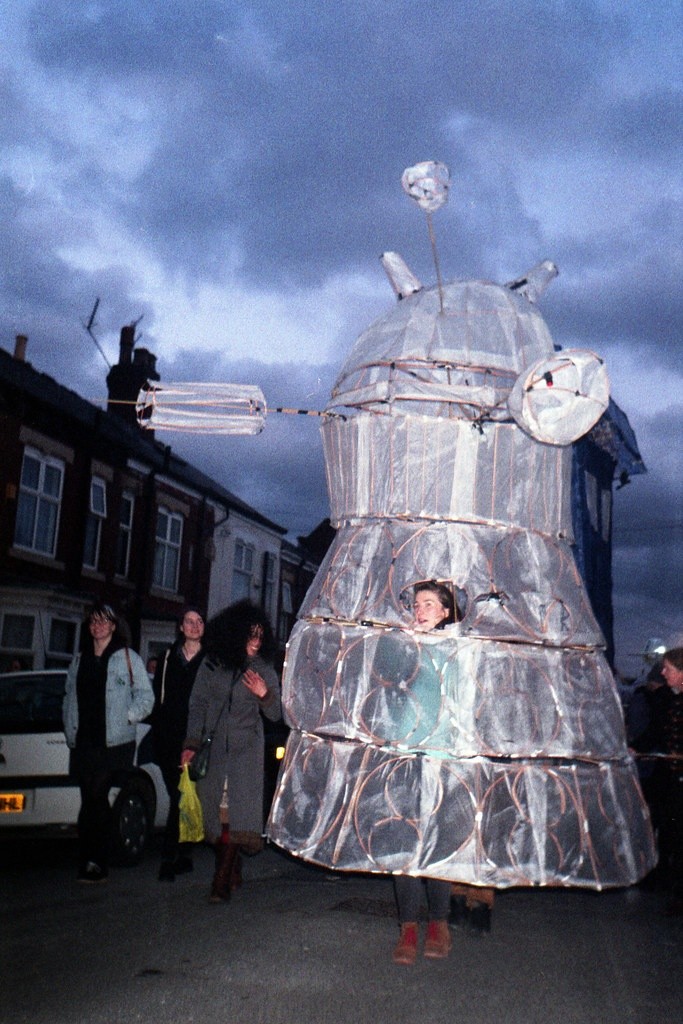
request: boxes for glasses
[88,616,113,626]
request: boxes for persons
[180,598,283,905]
[58,598,155,883]
[391,579,471,965]
[144,598,210,882]
[629,641,683,903]
[0,636,296,761]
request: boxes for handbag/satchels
[189,731,214,782]
[175,760,206,843]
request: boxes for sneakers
[446,894,468,933]
[389,921,417,965]
[466,903,492,939]
[422,919,453,958]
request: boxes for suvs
[0,670,169,869]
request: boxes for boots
[208,842,236,904]
[225,855,241,888]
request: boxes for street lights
[185,492,231,611]
